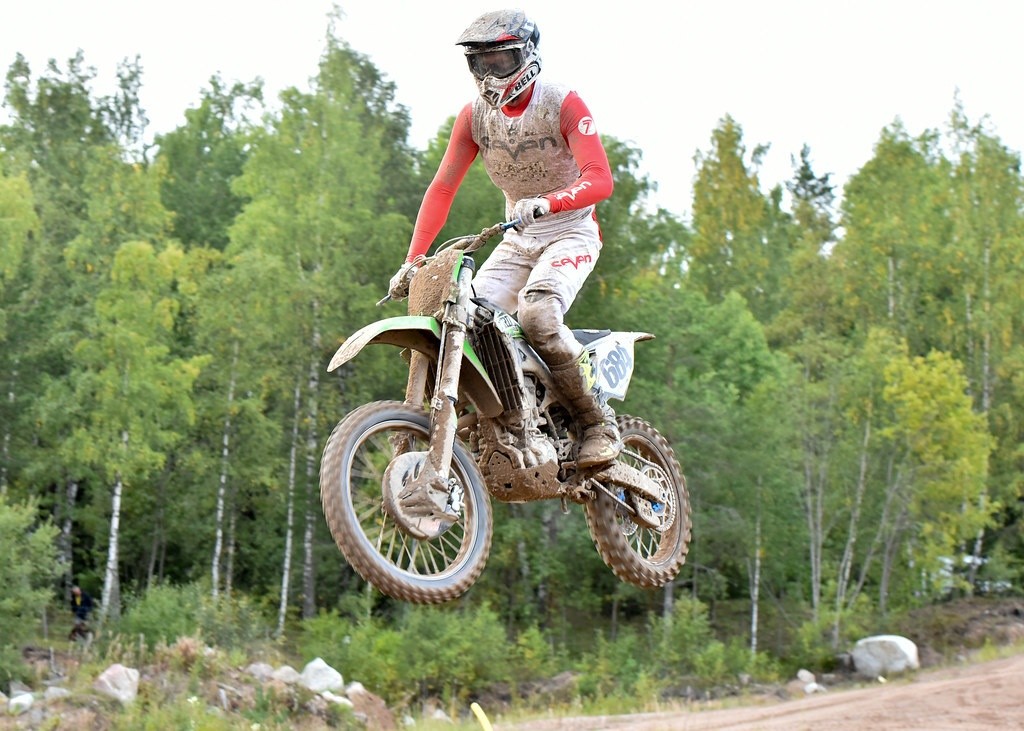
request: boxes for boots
[549,348,621,466]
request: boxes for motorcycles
[318,205,693,605]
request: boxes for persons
[386,6,625,466]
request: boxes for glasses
[465,25,539,80]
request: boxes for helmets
[455,9,539,111]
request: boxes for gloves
[388,263,421,302]
[511,196,549,237]
